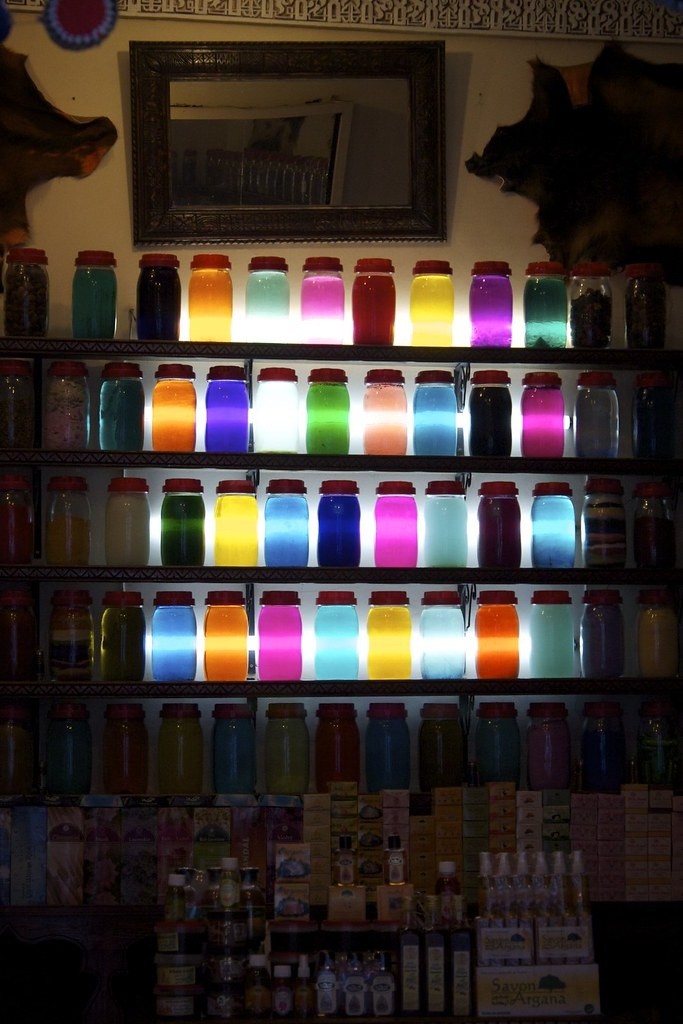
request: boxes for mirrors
[128,36,451,245]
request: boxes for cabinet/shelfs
[0,331,682,958]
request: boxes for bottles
[3,589,678,685]
[3,359,683,462]
[6,248,668,347]
[138,831,597,1022]
[6,704,683,792]
[3,477,681,574]
